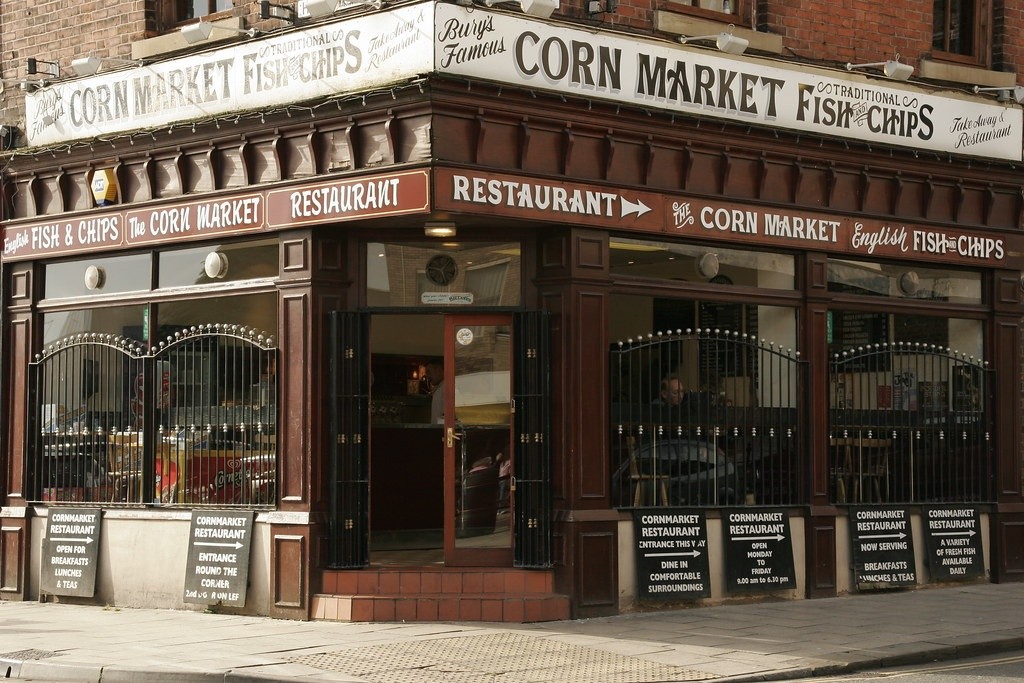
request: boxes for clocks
[425,254,459,286]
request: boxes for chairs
[829,437,853,502]
[628,436,671,505]
[854,437,891,501]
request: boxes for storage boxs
[385,395,432,423]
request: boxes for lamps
[424,222,456,238]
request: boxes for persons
[650,376,684,412]
